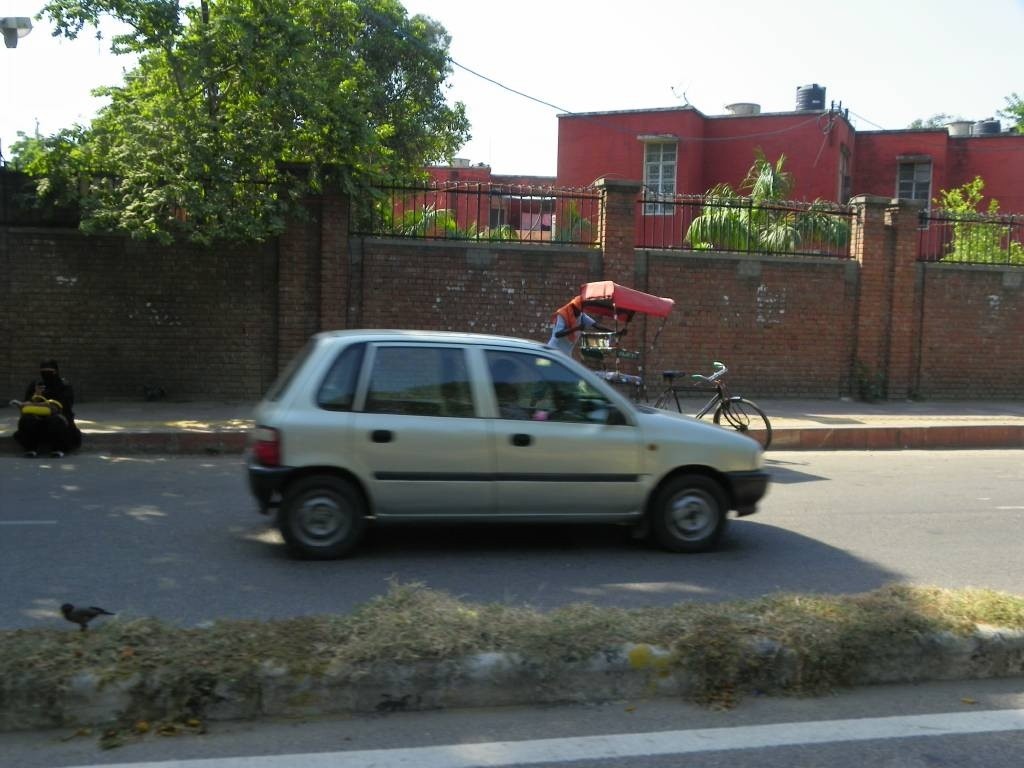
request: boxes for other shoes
[26,448,39,457]
[51,451,65,458]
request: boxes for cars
[249,329,770,563]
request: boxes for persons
[7,359,82,459]
[546,294,630,363]
[491,356,569,421]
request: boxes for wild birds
[55,603,116,632]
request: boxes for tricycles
[579,280,772,451]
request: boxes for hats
[40,358,57,372]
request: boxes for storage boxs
[580,332,616,347]
